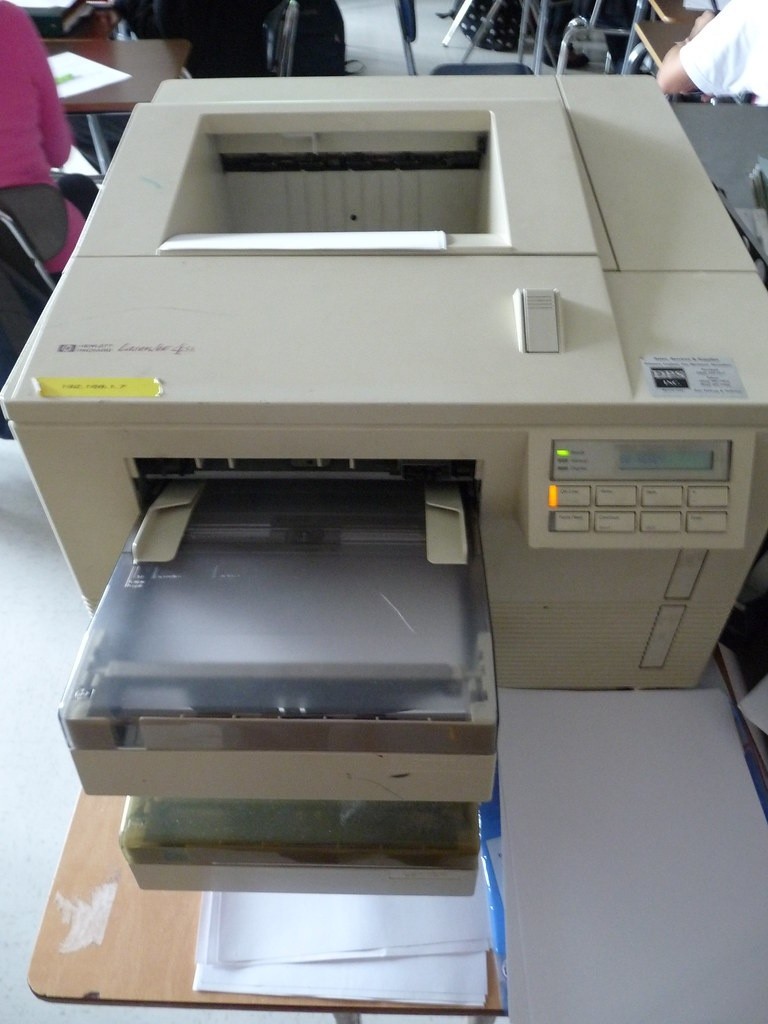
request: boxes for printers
[0,74,767,803]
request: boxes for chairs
[394,0,533,75]
[1,181,68,302]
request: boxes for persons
[0,0,97,287]
[656,0,768,105]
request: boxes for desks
[41,40,195,183]
[622,19,704,101]
[647,0,705,23]
